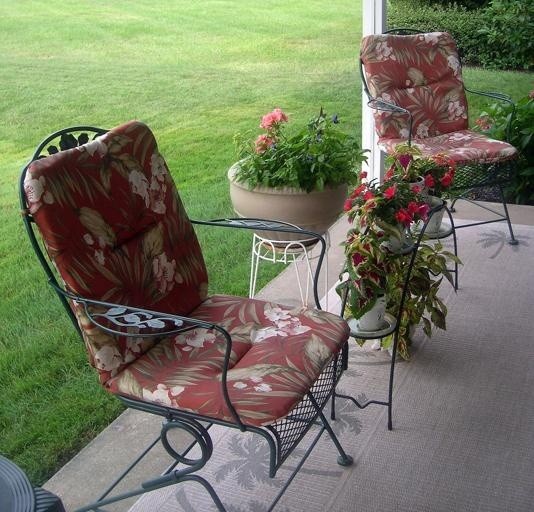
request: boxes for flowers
[340,143,459,361]
[241,113,371,192]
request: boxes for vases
[228,156,350,250]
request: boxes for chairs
[358,27,520,288]
[19,119,353,512]
